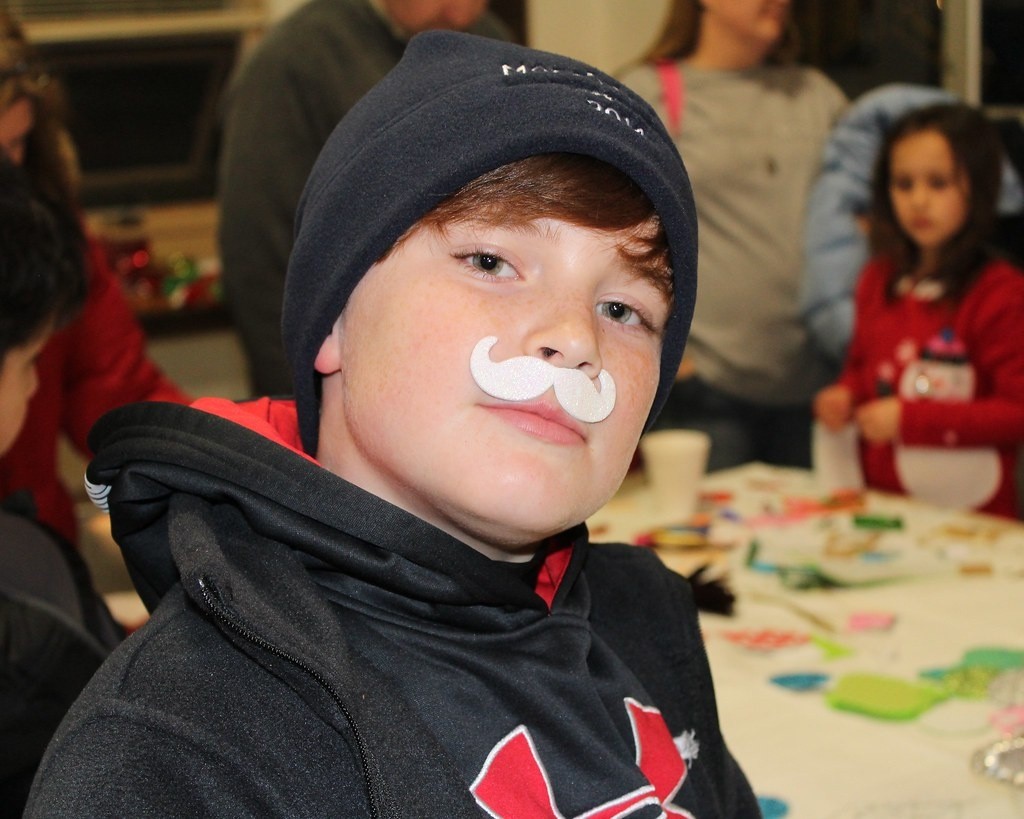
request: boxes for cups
[639,427,710,515]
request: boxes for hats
[283,29,698,459]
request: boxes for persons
[618,0,847,470]
[0,1,194,819]
[25,27,760,819]
[812,103,1024,518]
[215,0,510,399]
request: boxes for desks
[581,461,1024,819]
